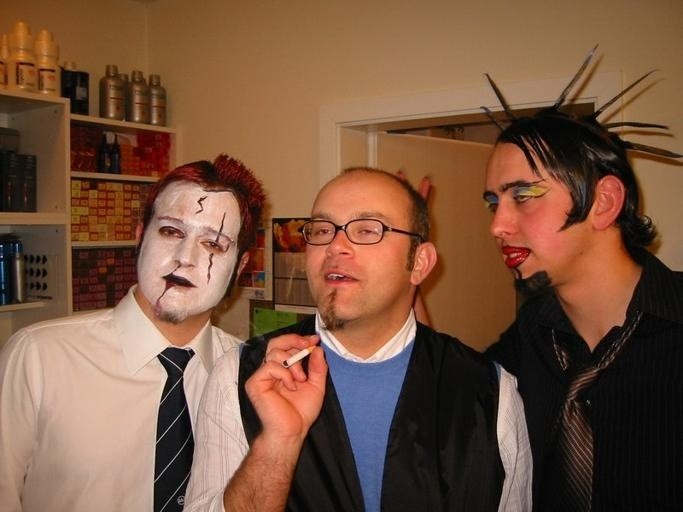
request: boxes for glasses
[298,219,425,245]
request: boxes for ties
[537,309,644,512]
[153,347,196,511]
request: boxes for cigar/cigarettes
[283,345,317,368]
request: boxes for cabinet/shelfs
[0,90,73,352]
[70,114,176,315]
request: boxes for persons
[183,167,533,512]
[480,44,683,512]
[0,153,265,512]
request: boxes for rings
[262,356,266,365]
